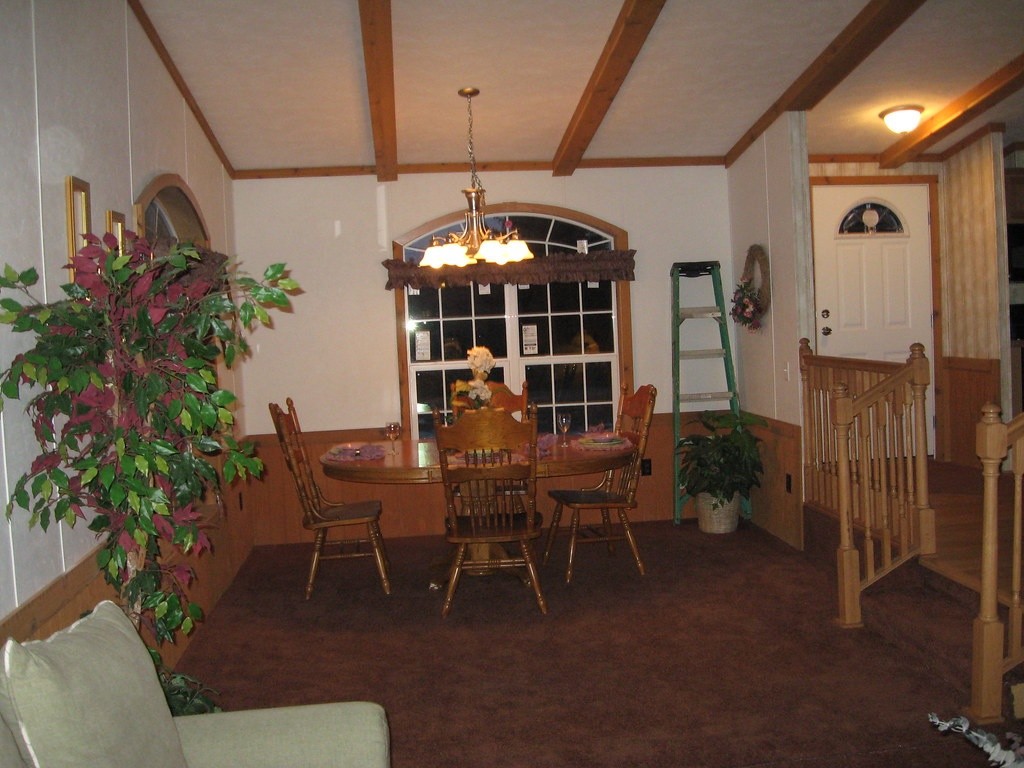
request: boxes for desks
[317,419,633,580]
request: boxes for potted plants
[676,412,772,537]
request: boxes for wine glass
[385,422,400,456]
[557,413,571,448]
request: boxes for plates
[579,437,624,445]
[465,451,506,461]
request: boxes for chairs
[429,404,553,616]
[539,381,659,582]
[452,376,533,429]
[269,395,397,604]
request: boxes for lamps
[418,85,536,274]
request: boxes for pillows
[0,597,191,767]
